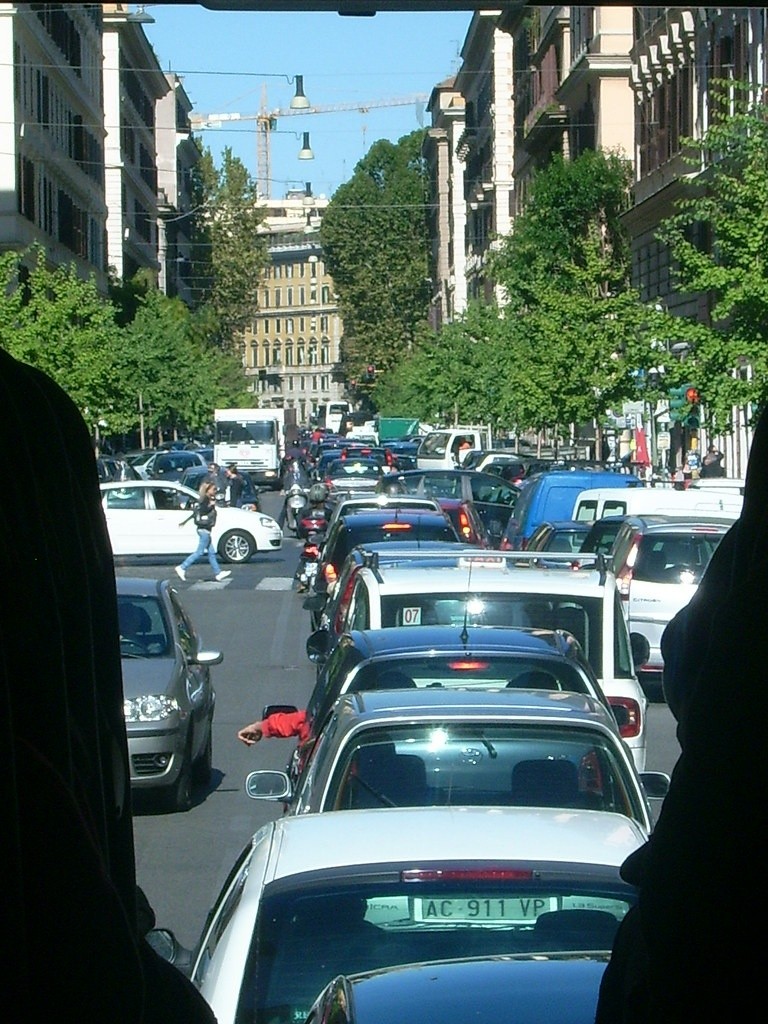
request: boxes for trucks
[416,431,591,475]
[213,407,298,489]
[346,417,420,440]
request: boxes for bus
[317,402,350,432]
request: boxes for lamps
[126,4,156,23]
[304,213,313,233]
[297,133,314,160]
[290,75,310,109]
[308,245,318,262]
[303,182,314,205]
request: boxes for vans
[502,472,647,554]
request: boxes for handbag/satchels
[194,499,216,527]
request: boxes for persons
[364,466,376,474]
[324,564,338,598]
[210,464,263,514]
[331,462,347,474]
[340,447,348,460]
[275,459,312,530]
[390,463,400,474]
[458,437,470,449]
[118,603,164,654]
[705,445,718,477]
[238,710,307,747]
[296,542,322,593]
[175,480,230,582]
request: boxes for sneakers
[175,566,187,581]
[215,570,231,580]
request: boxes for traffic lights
[367,365,374,382]
[684,388,700,429]
[669,386,684,422]
[350,380,357,391]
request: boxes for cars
[295,427,677,833]
[100,481,285,563]
[96,443,214,502]
[115,576,225,813]
[526,477,747,689]
[147,804,659,1023]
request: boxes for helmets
[309,484,326,503]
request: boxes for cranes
[187,81,439,201]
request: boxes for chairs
[512,760,580,805]
[370,670,416,690]
[531,908,617,957]
[358,753,427,810]
[506,671,560,690]
[135,606,165,645]
